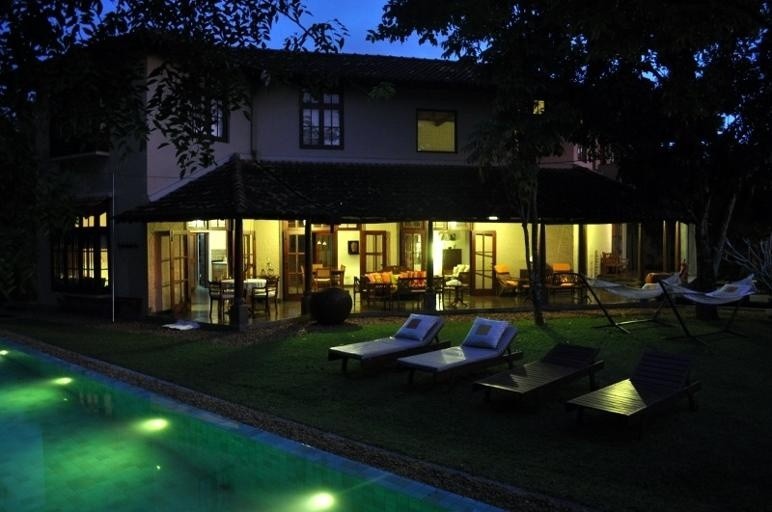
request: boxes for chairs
[550,263,576,296]
[328,314,452,379]
[569,350,708,445]
[352,263,470,311]
[205,275,279,324]
[398,317,523,402]
[467,340,605,428]
[492,264,521,297]
[299,264,345,293]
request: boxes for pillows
[393,314,440,341]
[462,317,512,349]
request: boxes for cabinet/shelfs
[441,249,461,287]
